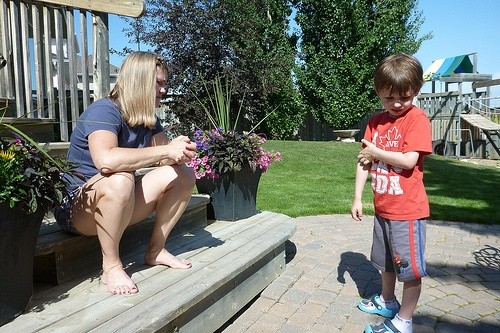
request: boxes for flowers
[0,94,120,216]
[175,70,283,186]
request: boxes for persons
[54,51,198,295]
[351,53,433,333]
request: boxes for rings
[181,154,184,157]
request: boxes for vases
[196,166,262,222]
[0,209,45,327]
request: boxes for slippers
[358,293,400,317]
[362,318,411,333]
